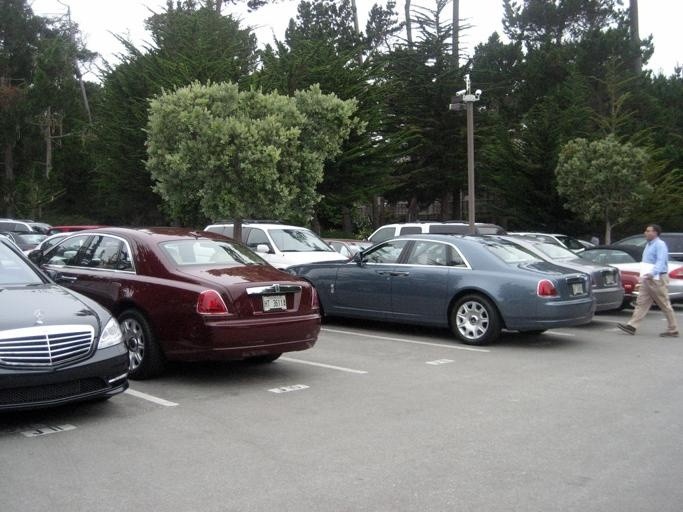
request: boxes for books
[637,261,660,282]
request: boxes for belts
[658,272,668,275]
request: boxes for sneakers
[616,322,637,336]
[659,327,680,338]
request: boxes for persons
[616,222,680,338]
